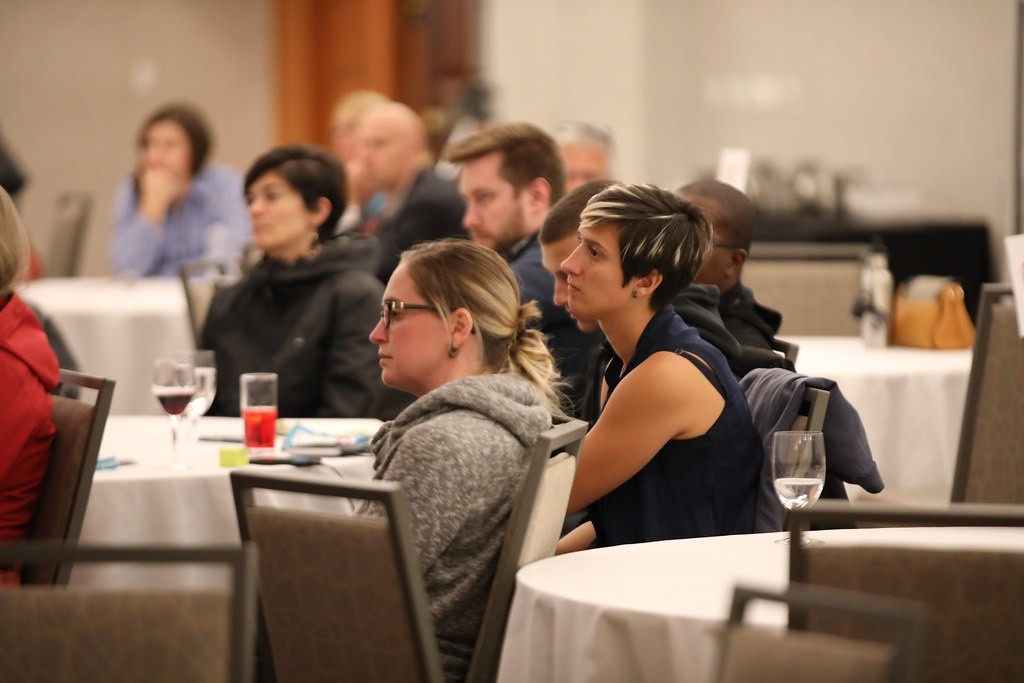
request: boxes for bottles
[860,254,893,350]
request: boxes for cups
[239,372,279,451]
[176,350,218,424]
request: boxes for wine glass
[151,359,197,448]
[770,430,824,547]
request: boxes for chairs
[0,192,1024,683]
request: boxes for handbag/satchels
[889,274,976,351]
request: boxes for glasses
[380,301,445,328]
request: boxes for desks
[69,414,384,587]
[13,277,240,416]
[774,336,977,508]
[496,527,1024,683]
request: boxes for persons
[113,100,254,279]
[540,179,623,431]
[352,238,561,683]
[448,121,604,413]
[555,183,765,557]
[0,183,62,589]
[676,178,782,379]
[328,88,470,285]
[199,141,385,419]
